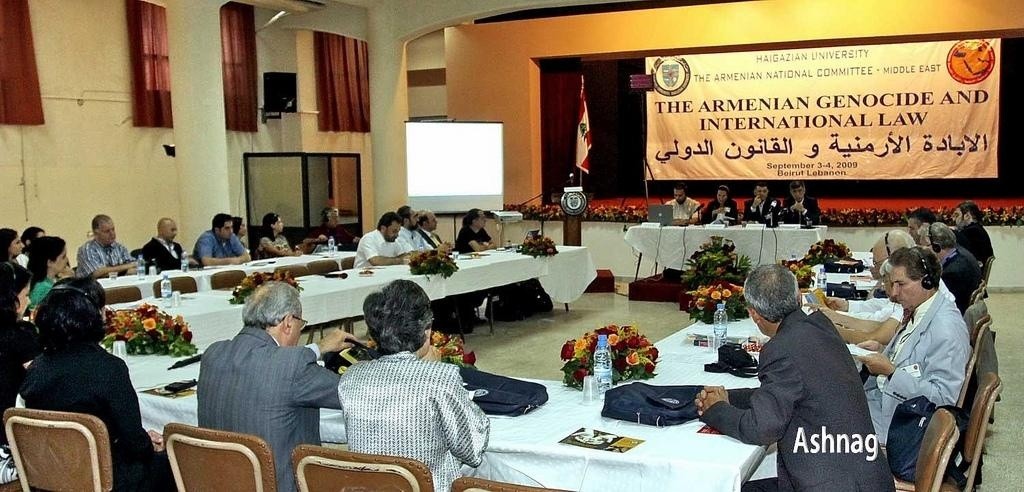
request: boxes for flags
[575,74,593,174]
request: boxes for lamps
[262,97,294,123]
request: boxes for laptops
[647,204,678,224]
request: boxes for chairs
[984,256,996,286]
[891,371,1005,491]
[956,313,993,411]
[969,279,987,307]
[913,408,960,492]
[1,407,115,492]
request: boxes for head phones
[926,234,942,254]
[920,268,933,290]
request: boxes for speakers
[263,68,300,118]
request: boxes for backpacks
[885,396,970,485]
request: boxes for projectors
[493,210,525,225]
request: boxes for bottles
[181,248,189,275]
[594,334,612,408]
[714,304,727,338]
[161,276,172,310]
[136,255,146,280]
[817,268,827,297]
[328,236,335,257]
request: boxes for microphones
[568,173,579,187]
[692,202,707,213]
[766,199,779,216]
[324,273,348,279]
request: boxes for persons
[778,180,821,226]
[0,227,76,314]
[197,280,359,492]
[75,215,139,280]
[700,185,739,227]
[822,200,994,347]
[742,182,782,228]
[190,203,303,266]
[663,182,703,226]
[0,262,42,485]
[355,205,454,268]
[338,279,491,492]
[853,246,972,450]
[13,277,177,492]
[693,263,896,492]
[302,206,364,254]
[453,209,498,254]
[138,218,199,275]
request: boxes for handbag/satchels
[601,379,714,425]
[823,258,864,274]
[449,365,553,419]
[703,343,759,378]
[826,281,856,299]
[324,337,376,378]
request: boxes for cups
[148,266,157,282]
[113,341,126,359]
[708,333,721,353]
[451,251,459,260]
[582,376,600,406]
[108,272,118,282]
[171,291,180,308]
